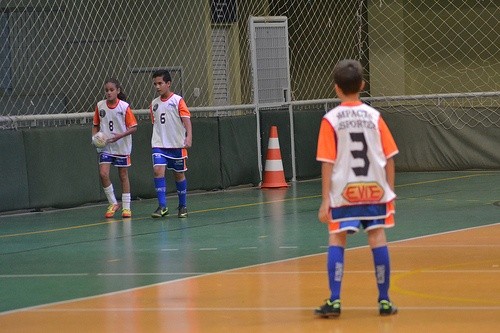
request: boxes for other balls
[93,132,108,147]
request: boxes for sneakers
[105,204,120,217]
[151,206,169,218]
[314,299,341,316]
[122,208,132,217]
[178,206,189,218]
[379,300,397,314]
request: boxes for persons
[314,60,399,318]
[150,70,193,217]
[92,79,138,217]
